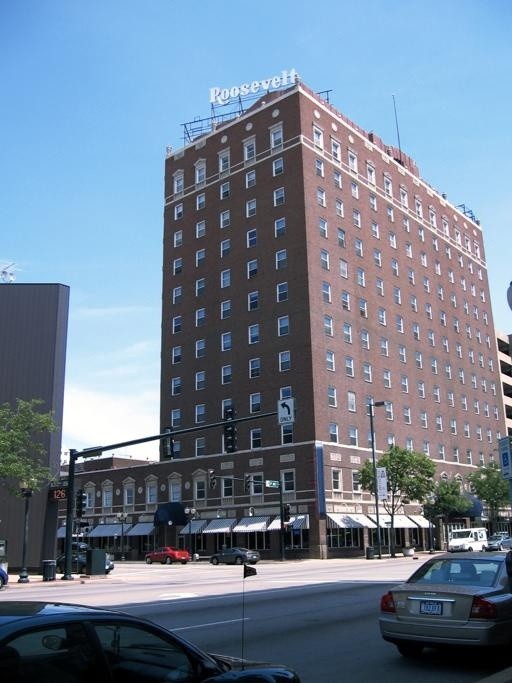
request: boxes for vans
[448,528,487,552]
[493,532,509,539]
[72,542,89,553]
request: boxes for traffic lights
[208,469,216,489]
[244,475,251,492]
[283,505,290,521]
[76,488,88,518]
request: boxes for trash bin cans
[366,546,375,559]
[41,559,57,580]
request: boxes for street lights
[369,402,385,558]
[18,476,38,583]
[117,513,128,561]
[248,507,257,549]
[217,510,227,550]
[421,497,435,549]
[184,504,196,562]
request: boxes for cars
[145,546,190,564]
[56,553,114,574]
[0,601,301,683]
[487,536,512,552]
[379,551,511,657]
[210,546,260,565]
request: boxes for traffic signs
[266,480,280,488]
[278,398,295,424]
